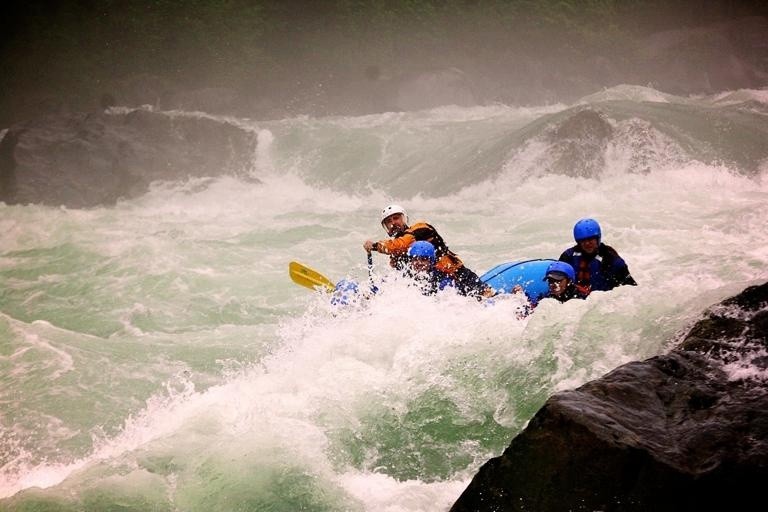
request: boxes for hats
[542,272,568,281]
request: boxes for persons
[558,219,638,291]
[400,241,488,302]
[363,206,498,302]
[513,260,594,322]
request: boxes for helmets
[545,261,576,282]
[378,205,410,238]
[573,218,601,246]
[407,240,436,265]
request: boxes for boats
[328,256,576,308]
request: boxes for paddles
[288,262,336,292]
